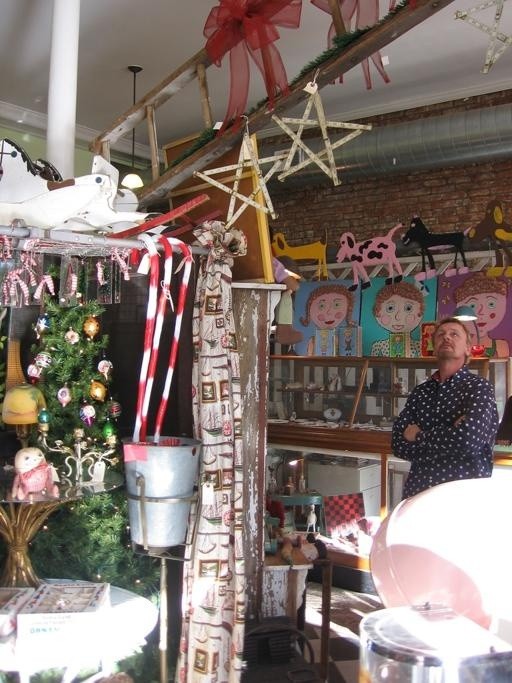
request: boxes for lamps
[119,58,147,190]
[3,381,45,451]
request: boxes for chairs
[241,622,314,666]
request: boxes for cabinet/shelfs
[263,353,512,598]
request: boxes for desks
[0,462,125,586]
[0,577,159,683]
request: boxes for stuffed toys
[11,444,61,500]
[264,523,328,568]
[266,220,305,346]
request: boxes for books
[420,322,441,357]
[389,333,412,359]
[314,329,337,358]
[337,326,363,358]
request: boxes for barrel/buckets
[123,435,200,546]
[261,565,313,617]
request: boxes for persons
[389,318,499,499]
